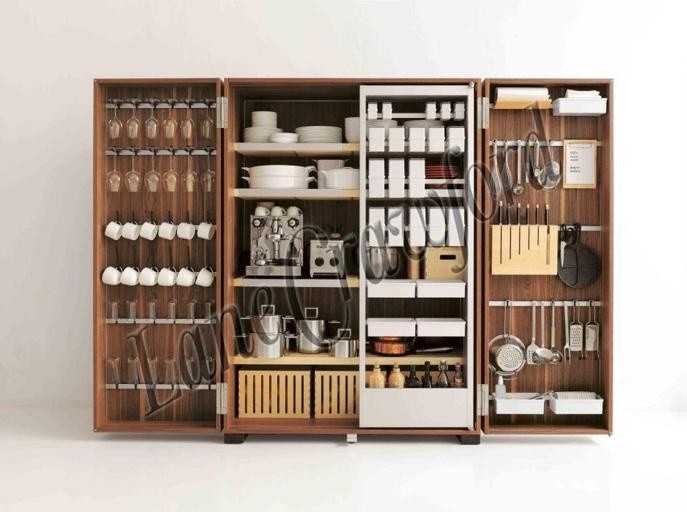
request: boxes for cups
[99,218,216,288]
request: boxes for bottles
[494,376,507,400]
[368,358,467,388]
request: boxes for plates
[242,110,466,144]
[423,164,463,189]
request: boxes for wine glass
[102,96,217,195]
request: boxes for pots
[231,305,408,359]
[556,222,602,289]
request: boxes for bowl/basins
[240,158,363,189]
